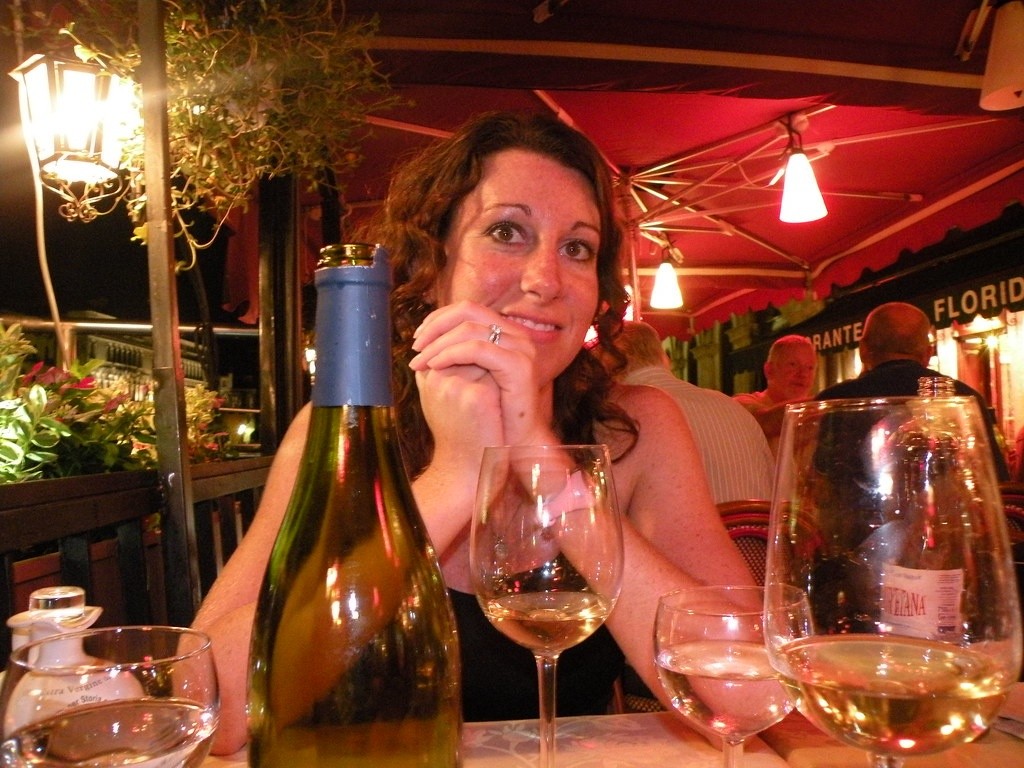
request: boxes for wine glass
[762,398,1022,768]
[469,446,624,768]
[653,586,814,768]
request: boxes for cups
[0,625,220,768]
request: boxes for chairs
[606,498,830,713]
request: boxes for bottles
[878,375,991,744]
[247,244,463,768]
[0,586,144,737]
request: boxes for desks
[198,710,1024,768]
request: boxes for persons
[168,111,794,758]
[726,330,820,506]
[810,300,1006,623]
[598,319,791,591]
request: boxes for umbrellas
[190,67,1024,341]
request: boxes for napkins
[994,680,1024,741]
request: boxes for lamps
[649,247,684,310]
[778,117,828,224]
[978,0,1024,112]
[5,30,139,222]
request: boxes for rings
[488,323,502,343]
